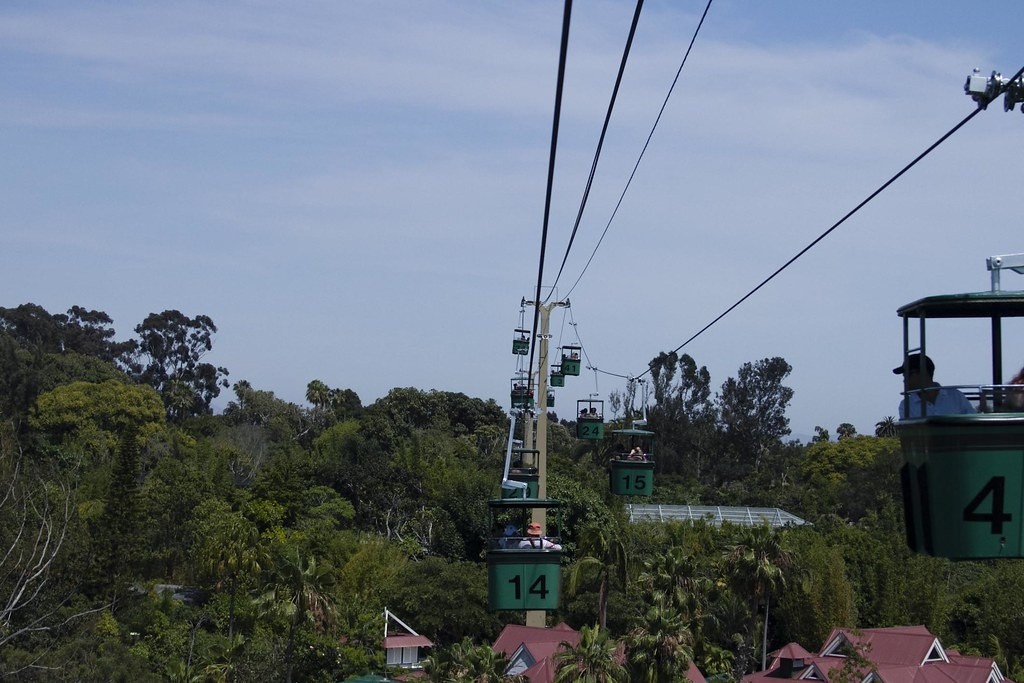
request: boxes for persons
[580,408,598,418]
[496,525,519,549]
[1003,367,1024,413]
[627,447,643,461]
[510,461,522,474]
[893,353,976,418]
[567,352,578,360]
[518,523,562,550]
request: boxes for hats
[528,523,542,535]
[893,353,936,376]
